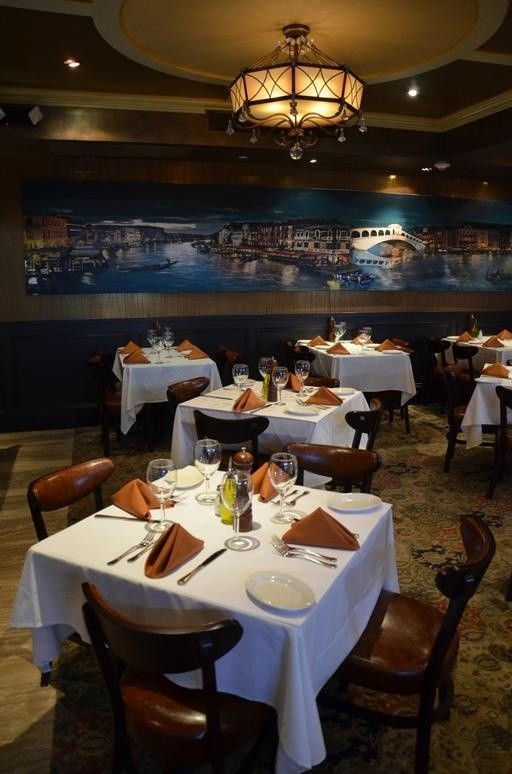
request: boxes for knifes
[178,548,227,585]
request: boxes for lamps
[229,23,366,159]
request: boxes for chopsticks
[202,392,233,402]
[250,402,271,412]
[95,513,161,523]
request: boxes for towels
[457,331,475,342]
[496,329,512,340]
[119,341,145,354]
[112,478,177,519]
[481,362,509,379]
[308,335,329,347]
[185,345,209,360]
[327,343,350,355]
[350,333,374,345]
[123,351,151,364]
[176,339,195,352]
[375,339,401,351]
[249,461,291,500]
[143,523,205,578]
[482,336,505,347]
[305,386,343,406]
[232,388,265,414]
[282,507,360,552]
[280,372,302,393]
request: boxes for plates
[287,405,320,415]
[326,492,381,511]
[365,342,382,346]
[332,387,355,394]
[164,467,204,488]
[382,349,403,355]
[314,344,330,349]
[246,570,316,611]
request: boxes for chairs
[193,410,269,476]
[426,338,451,371]
[29,458,114,686]
[221,351,236,386]
[488,386,512,499]
[90,355,155,456]
[97,349,117,393]
[316,513,496,774]
[81,581,279,773]
[391,338,409,347]
[345,398,384,492]
[283,443,382,492]
[288,341,293,372]
[167,376,210,434]
[390,347,416,434]
[293,347,339,387]
[445,380,499,472]
[210,345,225,369]
[453,343,481,406]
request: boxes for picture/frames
[20,174,512,296]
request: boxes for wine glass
[294,360,309,397]
[358,326,369,354]
[270,453,296,524]
[146,328,157,354]
[145,459,177,533]
[233,364,248,392]
[194,439,220,505]
[260,357,276,379]
[152,337,163,364]
[335,322,345,344]
[162,332,173,357]
[221,471,253,550]
[273,366,289,404]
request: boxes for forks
[127,541,157,563]
[286,490,308,505]
[108,529,156,565]
[272,488,298,505]
[270,534,337,570]
